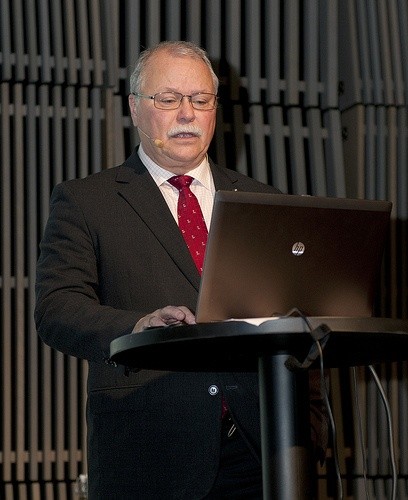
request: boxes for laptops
[196,189,393,319]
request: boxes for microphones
[136,127,163,148]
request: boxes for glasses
[131,91,220,111]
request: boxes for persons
[34,39,328,499]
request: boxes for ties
[166,175,209,278]
